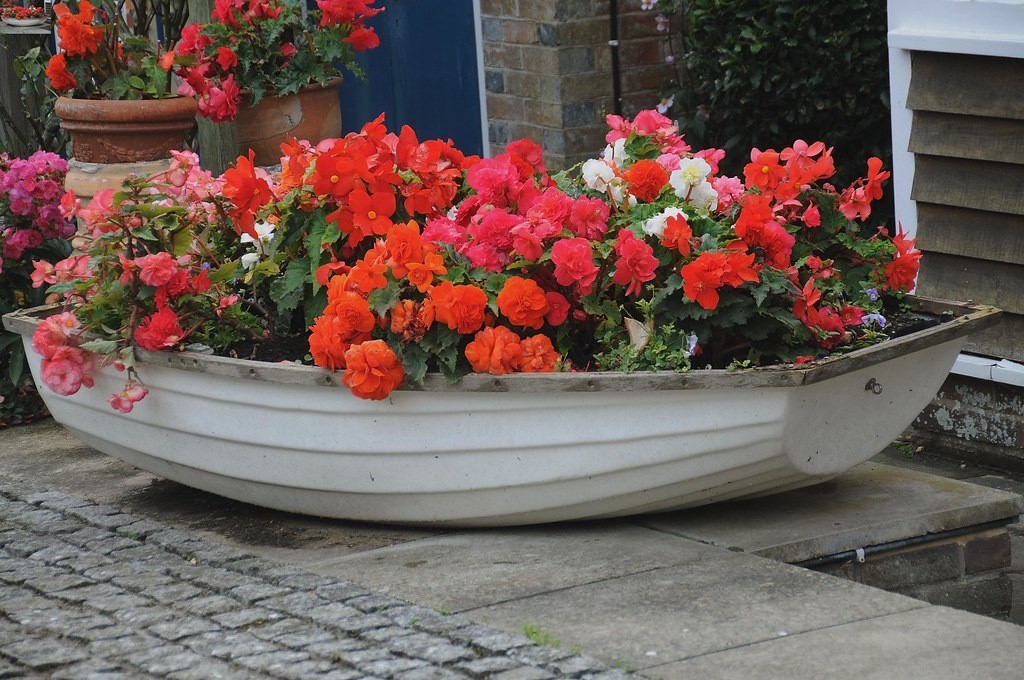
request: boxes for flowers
[0,5,45,20]
[158,1,386,119]
[32,91,925,415]
[43,2,192,102]
[1,145,82,315]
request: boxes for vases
[0,297,1007,529]
[1,17,47,27]
[63,156,174,243]
[225,72,345,168]
[51,94,199,164]
